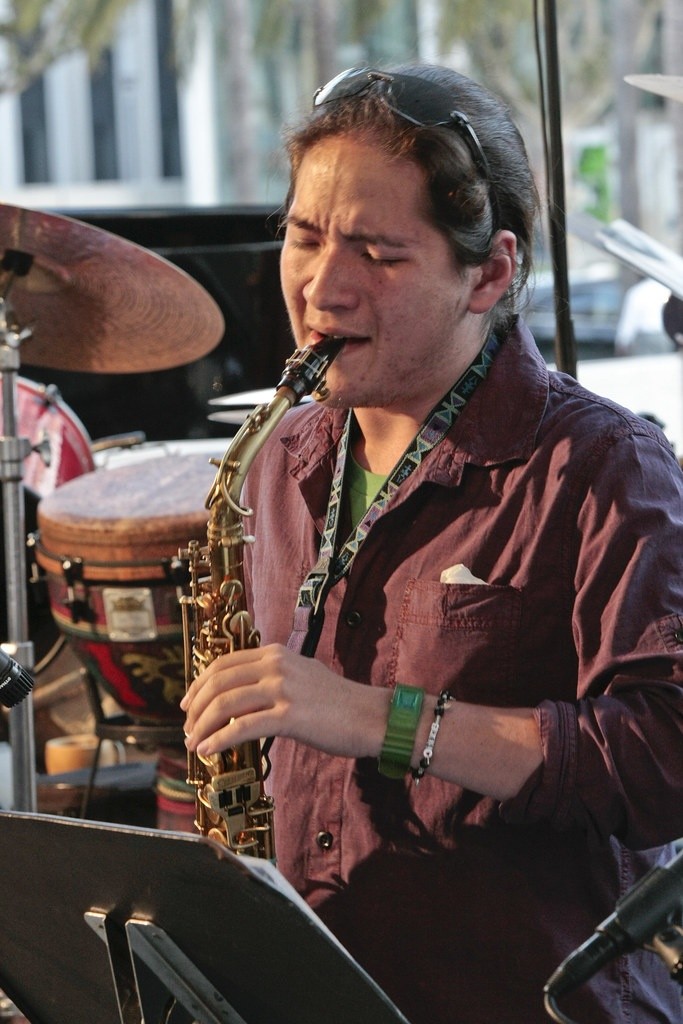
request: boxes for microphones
[542,851,682,995]
[0,648,33,707]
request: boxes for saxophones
[167,328,353,859]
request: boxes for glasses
[312,67,502,236]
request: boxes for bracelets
[411,688,451,783]
[378,682,424,778]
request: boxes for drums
[36,452,253,839]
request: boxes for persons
[176,62,683,1024]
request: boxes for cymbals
[206,387,316,424]
[1,200,224,379]
